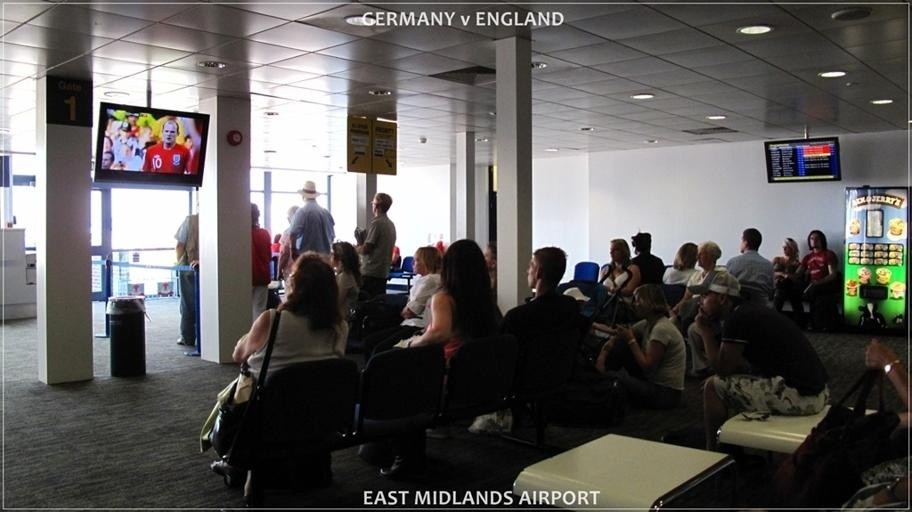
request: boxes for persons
[871,458,912,506]
[351,192,397,300]
[391,245,400,264]
[849,334,911,456]
[365,245,443,360]
[328,240,364,322]
[503,247,591,395]
[251,201,272,325]
[725,227,776,311]
[685,264,832,453]
[772,236,807,332]
[482,241,498,294]
[205,250,352,468]
[174,213,199,346]
[672,243,729,376]
[288,181,337,261]
[275,206,299,279]
[357,239,505,478]
[791,230,842,334]
[630,233,666,284]
[593,239,642,297]
[101,109,202,177]
[664,243,704,290]
[590,283,687,411]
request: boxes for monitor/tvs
[90,100,210,188]
[764,136,841,185]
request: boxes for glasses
[739,407,771,420]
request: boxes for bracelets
[408,339,413,347]
[887,477,901,498]
[881,359,899,375]
[626,337,637,346]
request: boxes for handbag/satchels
[367,293,408,314]
[755,403,900,511]
[266,287,281,308]
[208,403,267,473]
[536,367,626,428]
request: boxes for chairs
[386,256,414,293]
[363,344,445,444]
[209,355,360,503]
[574,261,599,283]
[445,334,520,414]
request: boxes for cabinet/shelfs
[0,228,39,319]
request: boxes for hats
[120,121,131,131]
[298,182,320,199]
[687,270,741,298]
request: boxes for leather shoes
[210,460,243,488]
[376,454,410,479]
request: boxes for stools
[510,432,737,511]
[716,402,833,454]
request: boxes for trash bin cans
[106,295,147,378]
